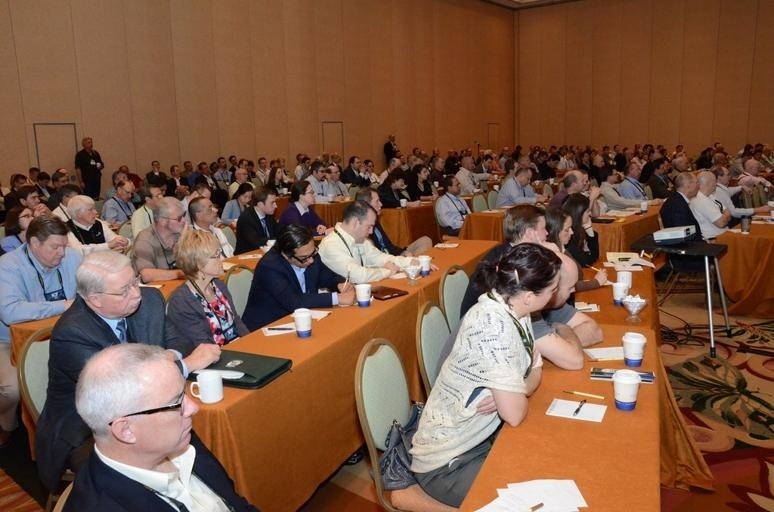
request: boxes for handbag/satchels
[370,400,424,491]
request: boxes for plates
[287,192,291,194]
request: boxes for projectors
[653,225,697,246]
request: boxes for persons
[0,134,774,512]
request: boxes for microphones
[473,140,482,147]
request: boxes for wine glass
[620,297,647,324]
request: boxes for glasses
[290,244,320,264]
[94,275,142,299]
[209,246,224,260]
[106,381,190,428]
[160,210,186,223]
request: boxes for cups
[190,371,223,404]
[353,283,371,308]
[294,308,312,337]
[493,175,498,181]
[327,194,335,203]
[433,182,438,188]
[611,369,641,409]
[534,177,559,206]
[283,188,287,196]
[402,255,431,287]
[492,184,498,191]
[621,331,647,366]
[634,200,648,216]
[741,215,752,234]
[767,200,774,221]
[612,271,632,307]
[399,199,407,208]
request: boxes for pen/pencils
[317,312,332,321]
[341,271,350,294]
[563,390,604,400]
[587,358,624,362]
[416,276,424,279]
[586,264,600,271]
[578,308,592,310]
[527,503,543,512]
[573,400,586,416]
[268,328,293,330]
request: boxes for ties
[260,218,268,237]
[115,319,129,346]
